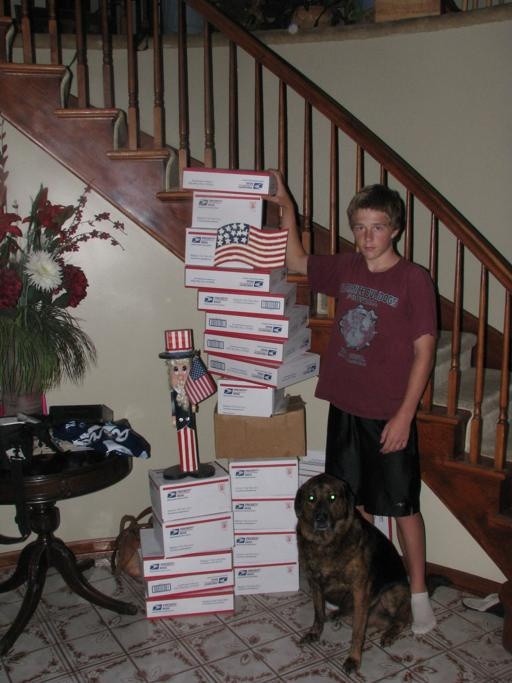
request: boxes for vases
[4,386,43,414]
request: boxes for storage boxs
[182,267,320,417]
[140,460,235,625]
[181,188,290,270]
[230,456,303,596]
[180,165,281,196]
[212,394,311,460]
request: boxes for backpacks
[110,506,154,575]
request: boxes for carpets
[59,65,511,462]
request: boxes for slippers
[462,593,504,618]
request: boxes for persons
[260,168,440,636]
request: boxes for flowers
[0,132,125,406]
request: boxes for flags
[184,355,218,404]
[213,222,289,268]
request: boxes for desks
[2,414,136,659]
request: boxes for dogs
[294,472,414,675]
